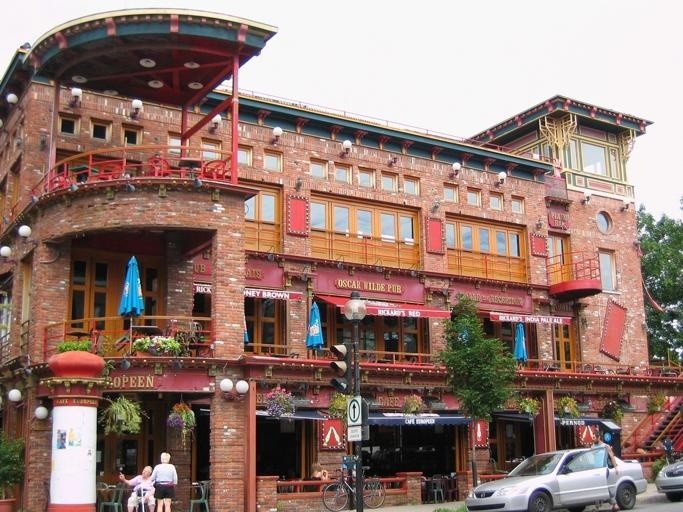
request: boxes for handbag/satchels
[137,489,147,497]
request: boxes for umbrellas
[514,323,528,363]
[118,255,145,354]
[306,301,324,359]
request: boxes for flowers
[167,393,197,439]
[645,393,664,414]
[134,335,182,357]
[516,398,540,420]
[603,400,626,420]
[557,398,579,419]
[264,384,297,423]
[403,394,423,414]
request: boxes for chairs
[145,155,171,176]
[421,474,458,503]
[190,480,211,512]
[204,158,226,180]
[97,481,126,512]
[330,344,678,377]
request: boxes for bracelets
[614,463,618,468]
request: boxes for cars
[655,459,683,502]
[465,443,648,512]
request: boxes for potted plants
[98,395,149,436]
[1,429,23,512]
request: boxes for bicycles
[323,469,386,512]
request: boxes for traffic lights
[330,342,352,395]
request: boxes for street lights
[344,291,367,512]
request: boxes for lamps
[210,114,221,134]
[124,174,136,194]
[219,378,248,403]
[17,225,35,244]
[130,99,144,118]
[7,389,24,409]
[69,180,80,194]
[0,94,18,130]
[30,188,43,203]
[449,162,462,179]
[272,127,283,143]
[620,197,631,212]
[34,398,49,421]
[495,172,508,187]
[1,246,16,263]
[339,139,352,158]
[582,190,591,204]
[70,87,84,106]
[191,165,204,188]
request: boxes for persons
[663,436,673,457]
[150,452,177,512]
[587,425,621,512]
[119,466,156,512]
[310,462,328,492]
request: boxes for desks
[113,326,160,356]
[69,165,100,182]
